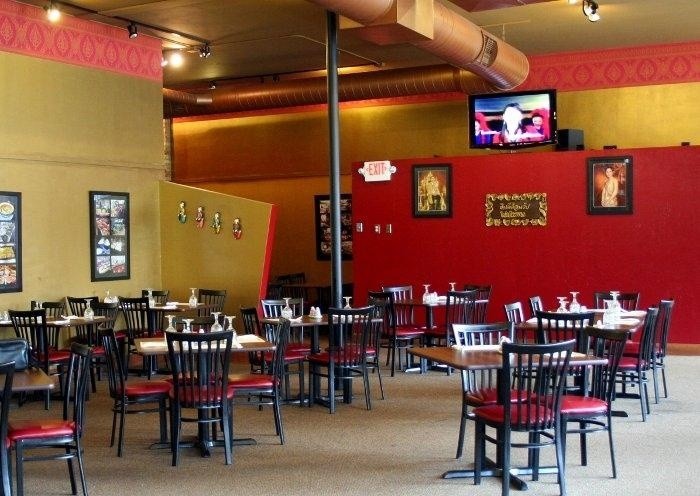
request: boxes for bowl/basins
[0,202,14,218]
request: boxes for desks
[135,333,278,450]
[407,346,608,492]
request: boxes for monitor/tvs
[468,88,558,150]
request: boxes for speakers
[556,128,584,150]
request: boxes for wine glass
[32,287,197,323]
[343,296,353,318]
[162,312,237,347]
[282,297,293,321]
[449,283,457,301]
[556,291,621,325]
[422,284,431,304]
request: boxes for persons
[532,113,550,141]
[473,119,492,145]
[492,103,545,144]
[601,167,618,206]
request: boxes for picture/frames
[413,163,451,218]
[588,157,633,213]
[89,191,130,282]
[0,191,22,293]
[314,193,352,261]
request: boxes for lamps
[582,0,600,22]
[44,0,211,59]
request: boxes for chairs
[369,284,492,377]
[226,318,289,445]
[99,328,172,456]
[535,325,629,479]
[0,271,235,409]
[10,341,92,496]
[504,290,648,402]
[167,332,234,466]
[3,361,11,496]
[622,300,673,404]
[240,273,385,405]
[473,338,577,495]
[307,305,374,414]
[603,307,659,422]
[453,323,537,459]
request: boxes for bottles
[308,306,322,319]
